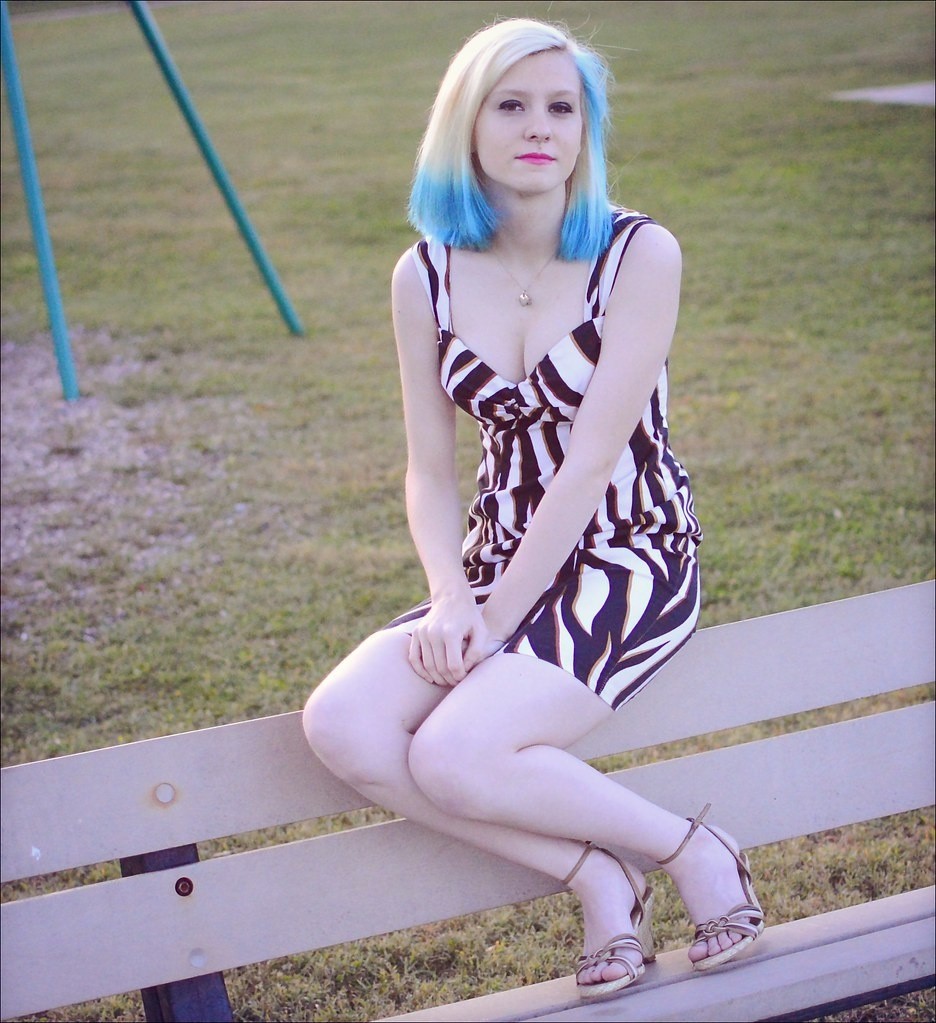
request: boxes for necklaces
[487,243,556,305]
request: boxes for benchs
[0,576,935,1022]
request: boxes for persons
[300,19,764,996]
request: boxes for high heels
[657,802,764,972]
[559,841,657,1001]
